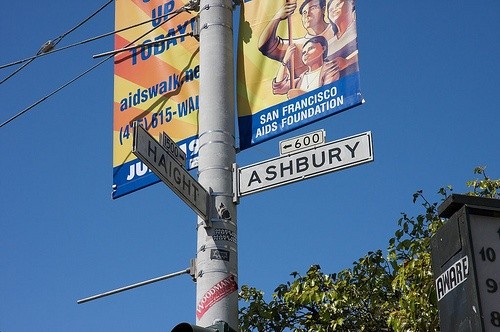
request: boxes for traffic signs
[233,131,374,203]
[132,121,212,228]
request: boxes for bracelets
[282,61,287,66]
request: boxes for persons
[271,37,341,100]
[258,0,333,78]
[322,0,358,77]
